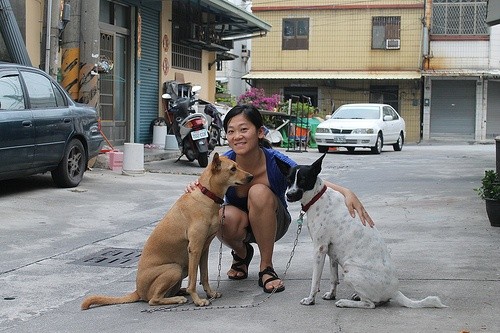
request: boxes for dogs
[80,152,255,310]
[273,151,448,309]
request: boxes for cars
[315,103,406,155]
[0,60,105,188]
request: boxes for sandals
[227,244,254,280]
[259,266,286,293]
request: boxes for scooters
[162,85,217,168]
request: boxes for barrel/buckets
[164,135,179,150]
[122,143,146,176]
[152,125,167,149]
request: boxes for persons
[184,104,374,295]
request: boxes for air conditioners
[242,49,251,57]
[386,39,401,50]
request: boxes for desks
[259,110,296,131]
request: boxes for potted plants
[473,170,500,227]
